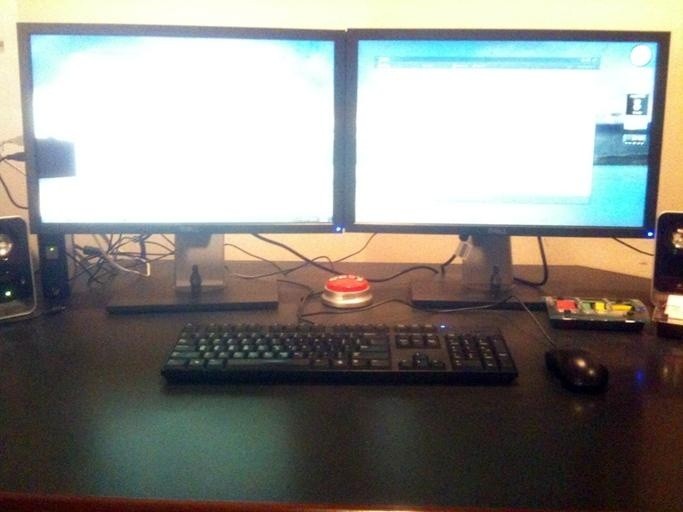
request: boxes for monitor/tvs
[16,21,344,316]
[346,27,672,310]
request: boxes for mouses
[541,342,610,399]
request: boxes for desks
[0,260,683,512]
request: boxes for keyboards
[162,320,521,388]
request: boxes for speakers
[650,211,683,308]
[0,215,39,323]
